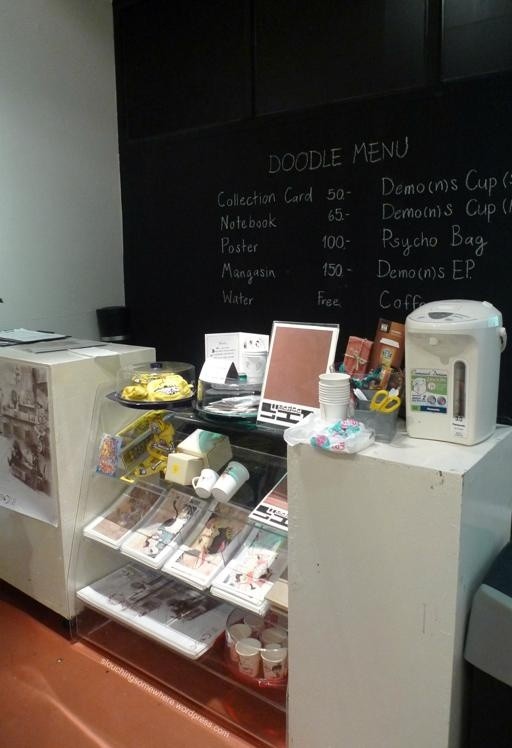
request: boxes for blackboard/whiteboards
[112,0,512,425]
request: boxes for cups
[229,623,253,663]
[244,615,265,641]
[210,461,250,504]
[192,468,220,499]
[261,628,287,648]
[242,349,267,385]
[318,372,351,420]
[261,643,288,681]
[235,638,262,677]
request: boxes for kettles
[403,299,507,447]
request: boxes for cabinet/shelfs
[71,396,287,747]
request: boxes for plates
[190,391,261,425]
[105,387,198,410]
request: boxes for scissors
[370,389,401,414]
